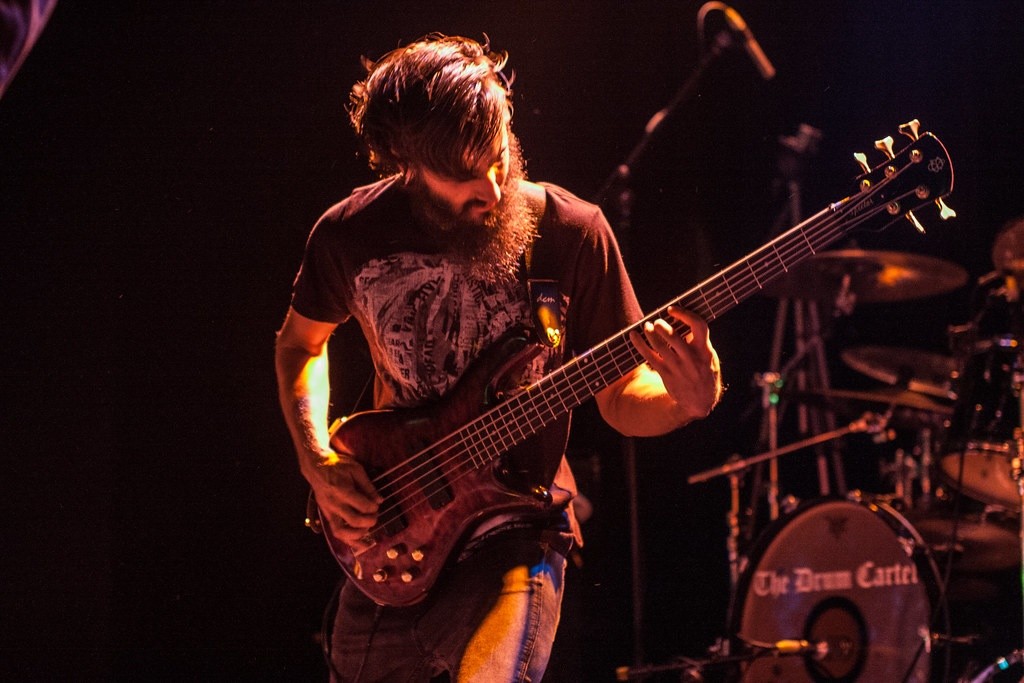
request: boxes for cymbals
[770,249,964,308]
[839,343,959,397]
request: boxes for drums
[934,333,1024,522]
[729,491,1023,683]
[896,420,1020,575]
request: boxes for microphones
[775,638,830,661]
[727,7,775,80]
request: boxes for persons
[275,33,724,683]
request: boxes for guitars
[310,116,956,607]
